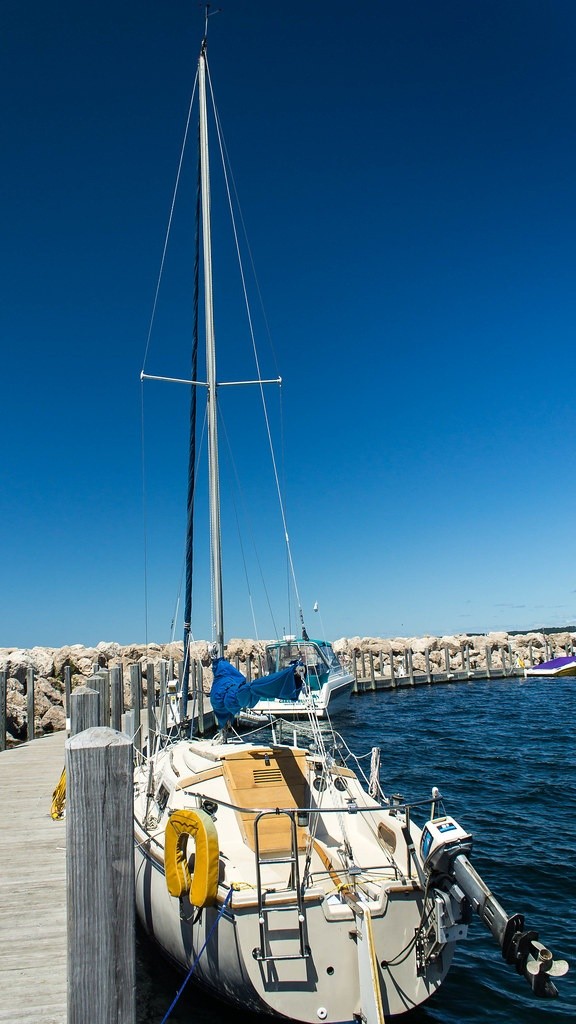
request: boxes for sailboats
[132,5,568,1024]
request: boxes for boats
[240,639,354,720]
[526,656,576,678]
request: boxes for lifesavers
[164,808,220,907]
[515,657,525,668]
